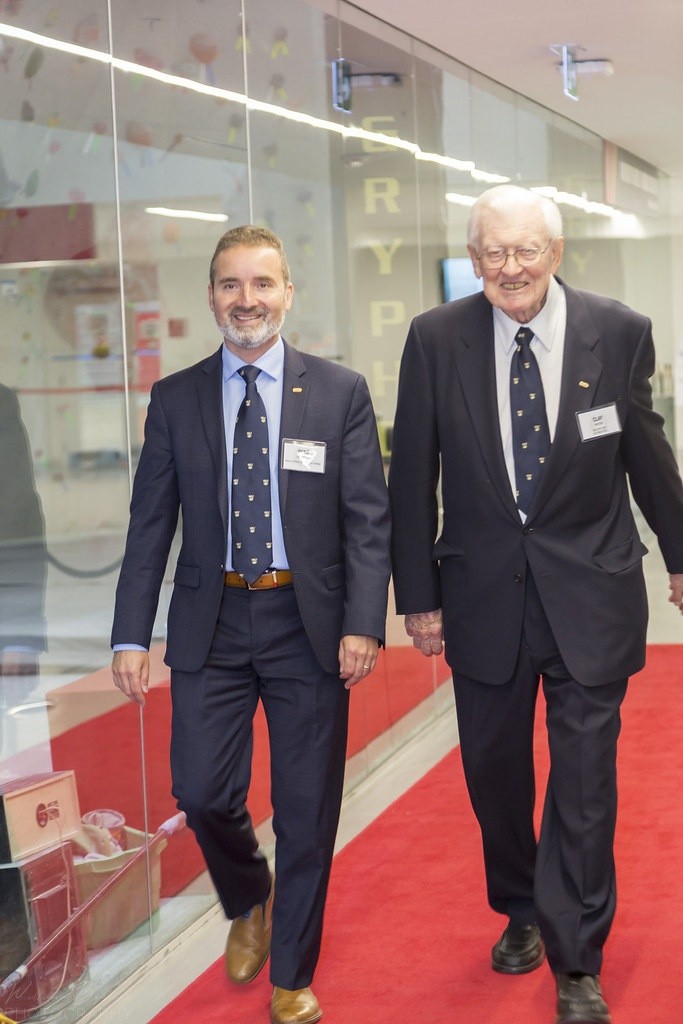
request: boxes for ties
[231,365,273,584]
[509,327,551,514]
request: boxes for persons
[0,383,51,721]
[389,180,683,1022]
[108,224,393,1024]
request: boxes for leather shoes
[492,915,545,975]
[270,985,322,1024]
[554,971,611,1024]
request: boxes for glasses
[475,238,552,270]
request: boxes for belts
[225,874,275,983]
[224,571,293,590]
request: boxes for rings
[363,665,370,668]
